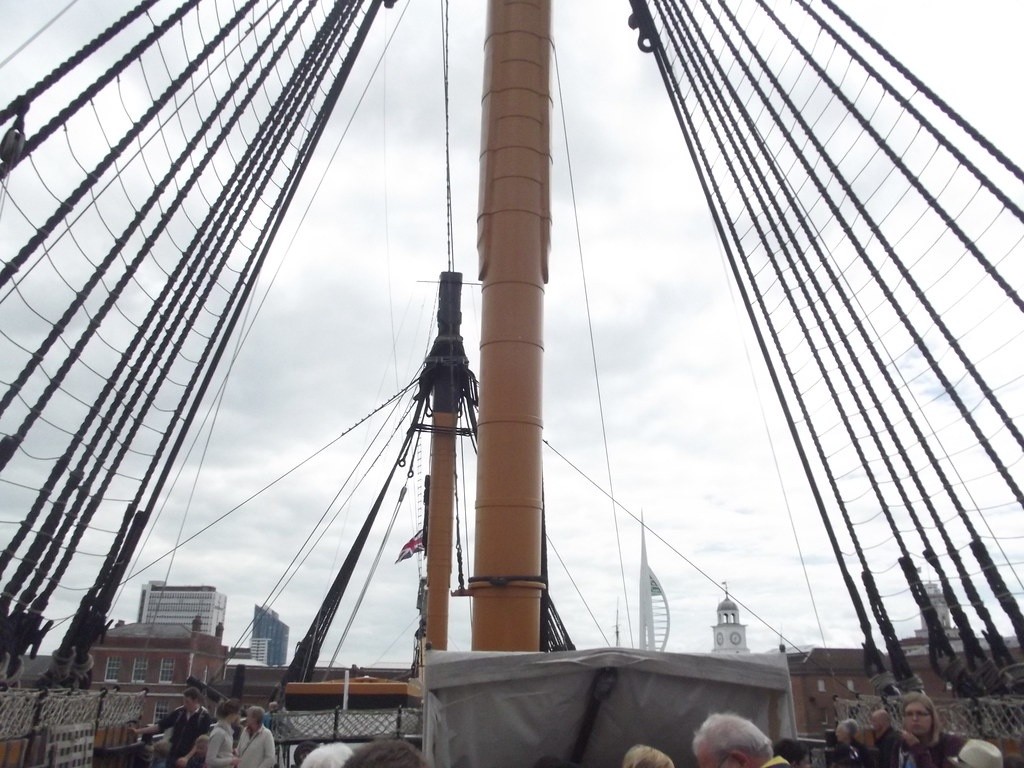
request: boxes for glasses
[719,752,730,767]
[906,709,926,717]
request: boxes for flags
[395,530,424,564]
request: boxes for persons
[831,692,1003,768]
[693,715,805,768]
[624,744,673,768]
[300,740,430,767]
[126,688,278,768]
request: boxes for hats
[946,739,1003,768]
